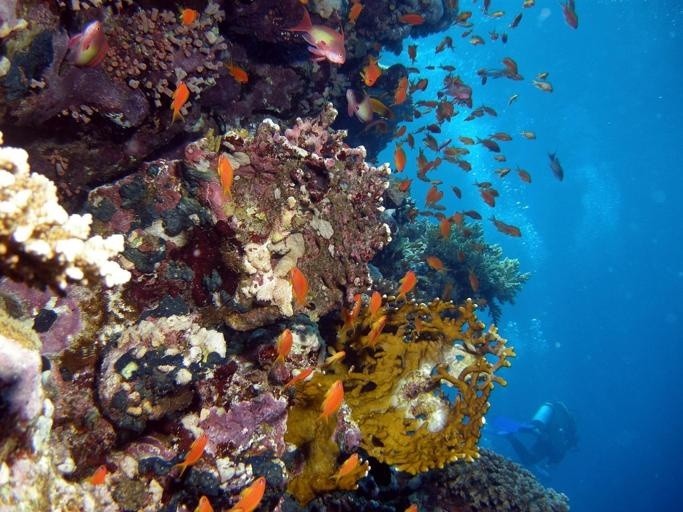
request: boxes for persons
[510,399,577,463]
